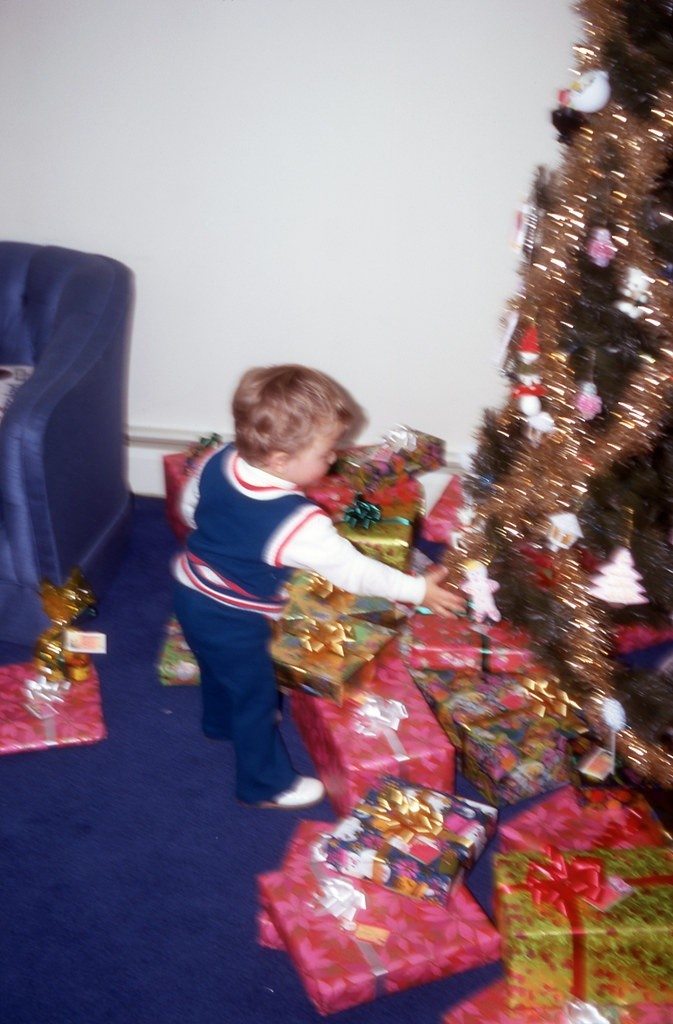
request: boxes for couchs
[0,241,132,647]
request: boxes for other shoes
[252,775,327,807]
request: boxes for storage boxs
[162,382,672,1023]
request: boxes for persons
[170,366,466,808]
